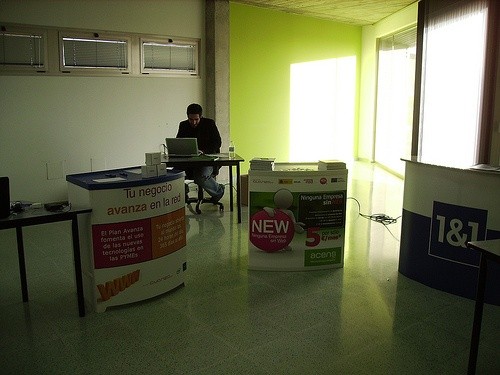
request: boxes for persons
[172,103,225,203]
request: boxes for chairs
[185,162,224,214]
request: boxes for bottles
[228,140,235,159]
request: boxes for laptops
[166,138,201,156]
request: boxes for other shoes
[211,184,225,205]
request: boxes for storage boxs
[242,175,249,206]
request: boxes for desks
[66,165,187,312]
[246,164,348,271]
[398,156,500,306]
[146,151,244,224]
[1,202,92,318]
[467,239,500,375]
